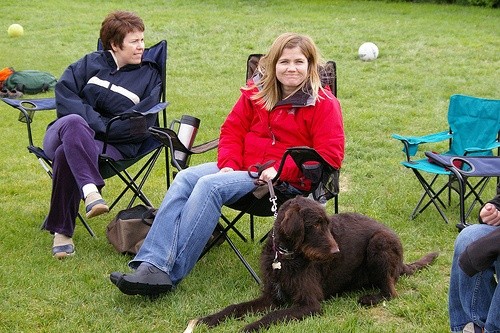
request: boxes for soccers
[359,41,379,60]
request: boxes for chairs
[424,152,500,232]
[1,38,171,237]
[148,53,341,287]
[391,94,500,224]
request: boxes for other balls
[8,23,23,40]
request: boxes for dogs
[187,195,439,333]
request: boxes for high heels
[110,272,120,284]
[117,264,172,294]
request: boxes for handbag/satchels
[107,204,159,252]
[7,70,57,94]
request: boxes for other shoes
[53,234,75,257]
[0,89,10,98]
[84,191,109,219]
[9,90,23,98]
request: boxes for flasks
[170,115,201,168]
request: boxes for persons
[109,33,345,296]
[41,10,163,258]
[449,177,500,333]
